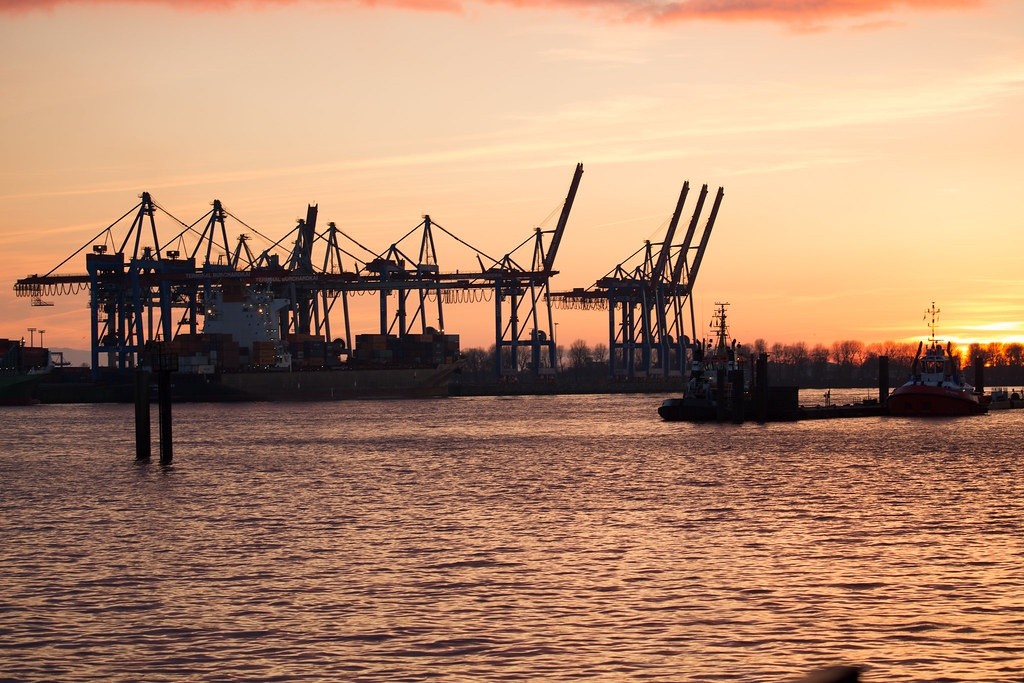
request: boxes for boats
[658,301,798,421]
[220,358,469,402]
[885,298,988,415]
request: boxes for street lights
[554,323,559,345]
[27,328,36,347]
[38,330,45,349]
